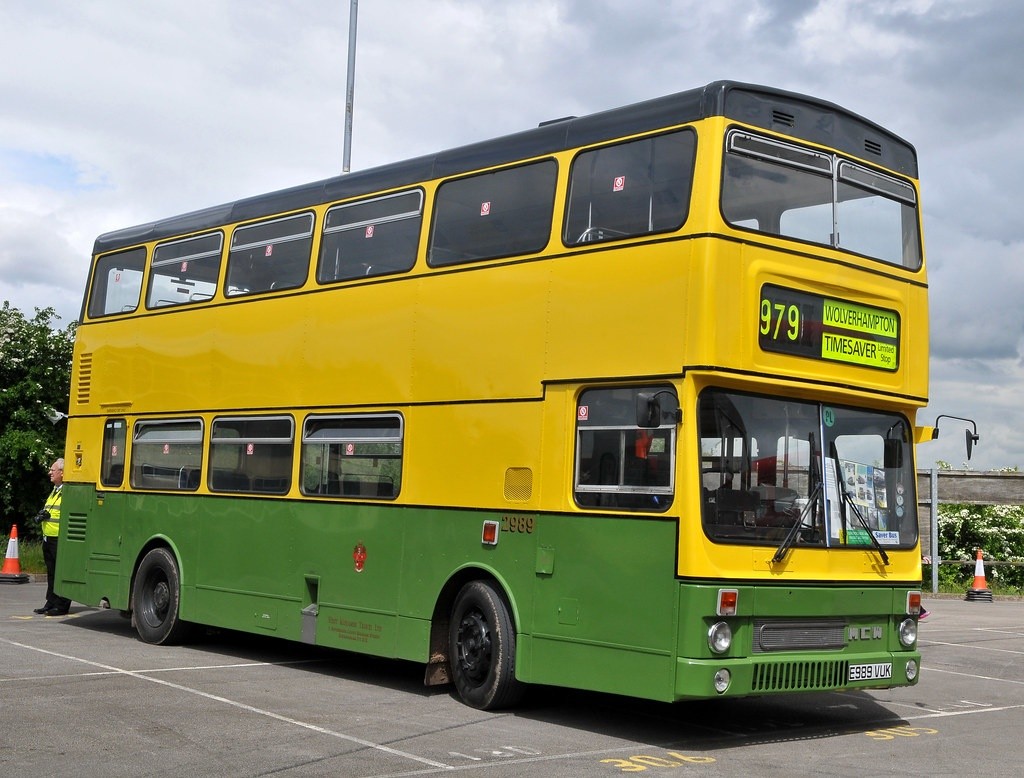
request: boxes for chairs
[575,226,629,243]
[121,227,400,316]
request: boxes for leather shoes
[45,607,69,616]
[34,604,54,614]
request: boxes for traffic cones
[0,523,30,578]
[965,549,993,592]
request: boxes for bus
[53,79,979,714]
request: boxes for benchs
[109,464,395,498]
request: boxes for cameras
[34,510,51,525]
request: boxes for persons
[34,458,72,616]
[104,465,121,484]
